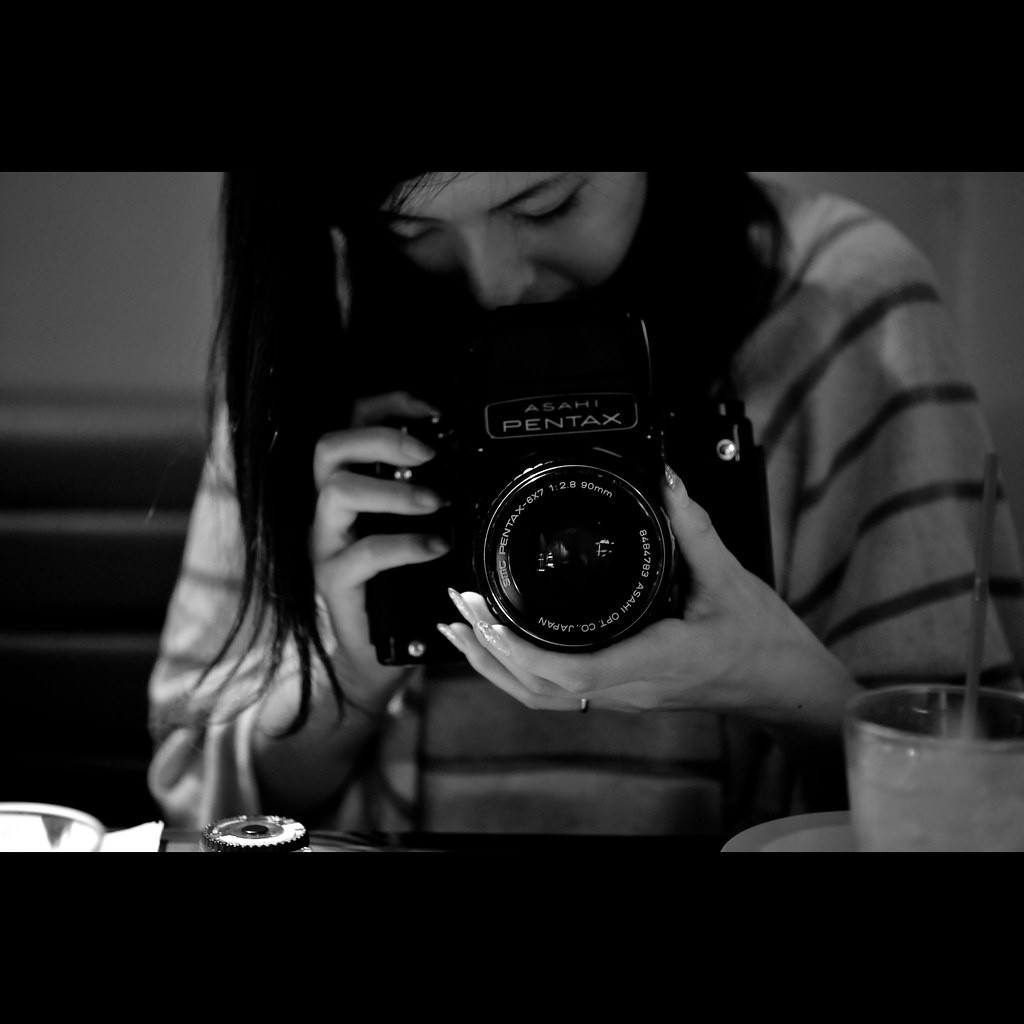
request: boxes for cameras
[354,304,773,666]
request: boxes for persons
[145,171,1013,854]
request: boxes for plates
[719,811,852,852]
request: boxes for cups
[842,682,1024,852]
[0,801,104,852]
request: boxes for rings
[579,698,589,713]
